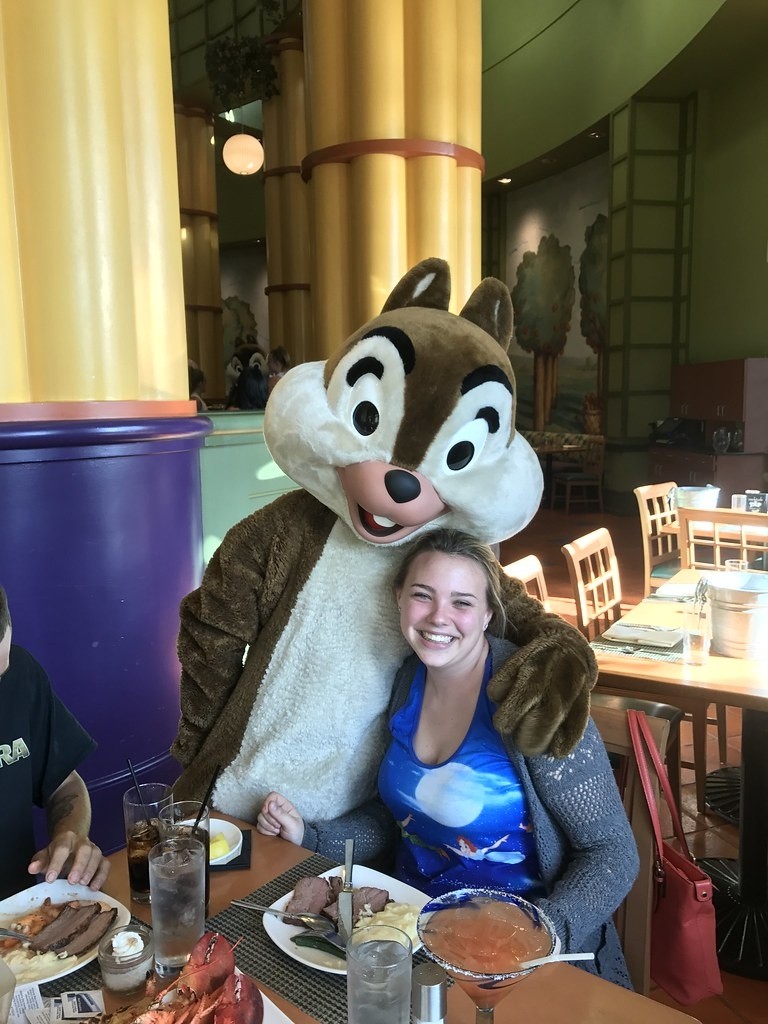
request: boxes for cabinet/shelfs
[669,357,768,457]
[645,444,768,550]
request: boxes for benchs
[515,430,607,508]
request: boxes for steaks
[322,886,389,932]
[282,875,343,927]
[54,907,118,959]
[28,903,102,954]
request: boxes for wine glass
[417,889,557,1024]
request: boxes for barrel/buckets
[703,571,768,659]
[672,484,721,528]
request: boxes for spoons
[230,899,336,933]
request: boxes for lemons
[210,831,229,859]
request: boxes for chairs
[501,554,684,839]
[677,506,768,574]
[550,435,607,516]
[632,483,714,598]
[588,705,670,1000]
[561,527,727,814]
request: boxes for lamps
[222,106,265,176]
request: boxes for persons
[267,344,291,394]
[188,359,210,413]
[224,367,269,412]
[256,528,639,990]
[0,583,110,902]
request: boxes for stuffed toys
[169,256,599,823]
[223,334,269,385]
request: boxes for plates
[263,864,433,975]
[563,445,581,448]
[133,966,294,1024]
[0,880,131,988]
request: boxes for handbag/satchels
[624,709,725,1003]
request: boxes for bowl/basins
[175,818,242,865]
[98,925,154,996]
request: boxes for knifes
[339,839,352,940]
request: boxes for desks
[657,519,768,572]
[589,568,768,981]
[531,444,586,510]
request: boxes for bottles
[731,424,743,452]
[713,422,730,452]
[411,964,446,1024]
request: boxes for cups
[725,559,748,571]
[149,839,205,978]
[684,626,707,664]
[159,801,210,920]
[732,495,746,513]
[346,925,412,1024]
[124,784,174,904]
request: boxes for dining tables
[40,808,702,1024]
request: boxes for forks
[296,931,347,953]
